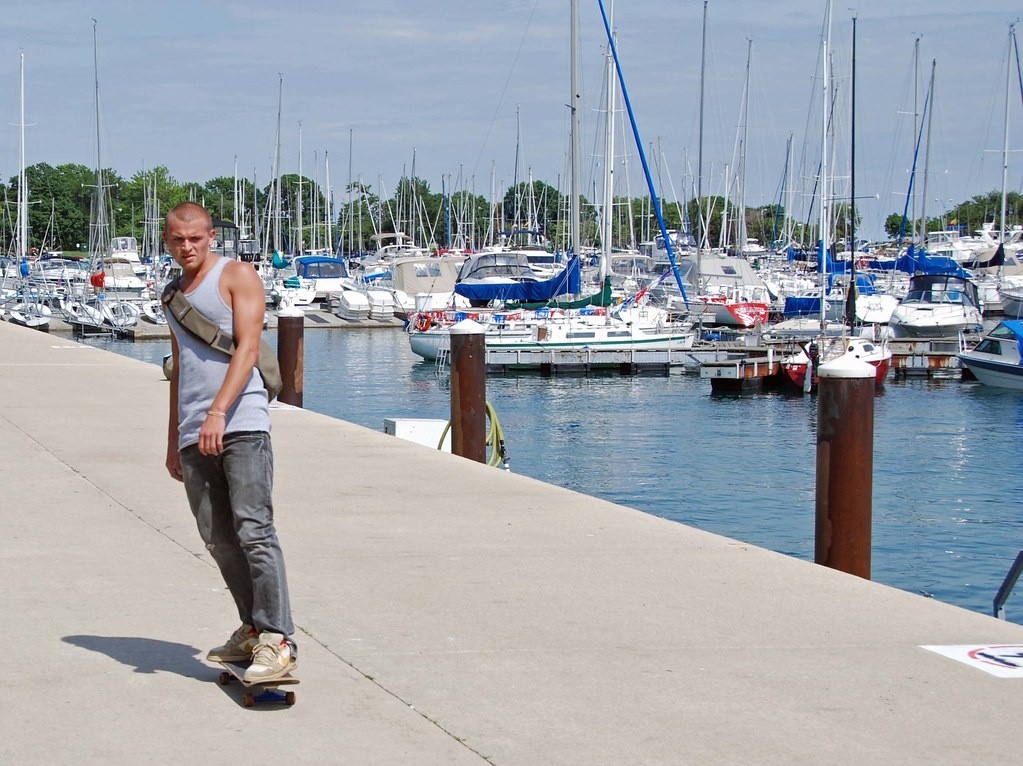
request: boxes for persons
[161,202,298,682]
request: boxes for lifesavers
[431,310,441,321]
[149,280,155,289]
[29,247,38,255]
[415,313,432,331]
[857,260,870,270]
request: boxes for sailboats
[0,1,1023,400]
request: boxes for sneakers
[243,631,297,682]
[206,623,260,661]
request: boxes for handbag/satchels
[254,335,282,403]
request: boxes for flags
[949,219,957,226]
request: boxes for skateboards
[218,658,301,707]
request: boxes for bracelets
[208,411,227,417]
